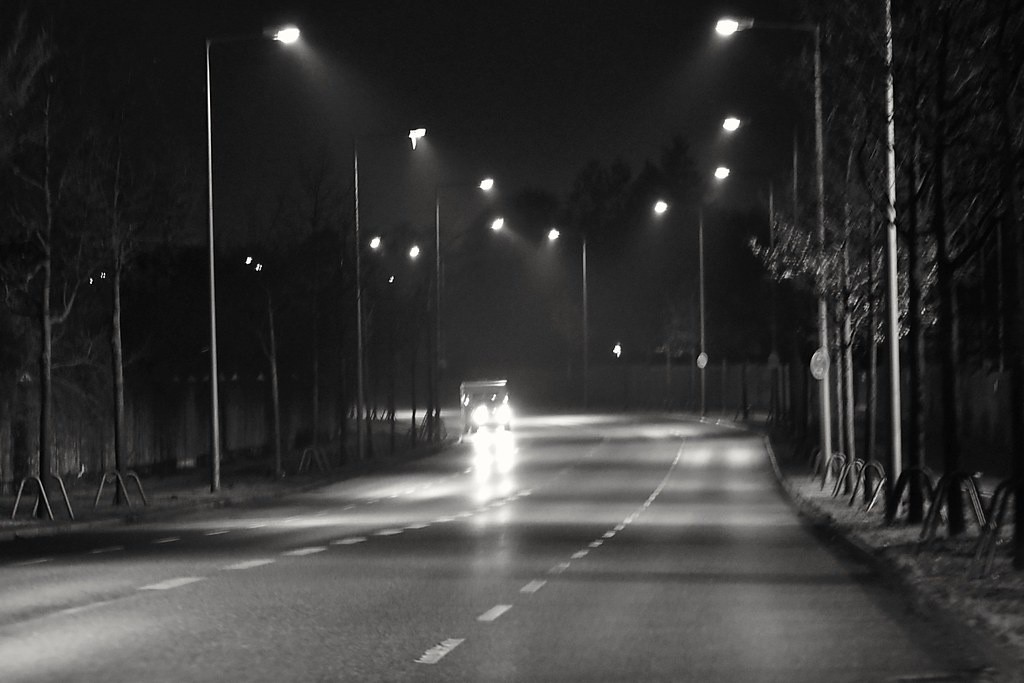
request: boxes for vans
[459,380,512,435]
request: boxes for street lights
[651,14,836,495]
[201,20,300,493]
[353,124,428,462]
[432,165,597,408]
